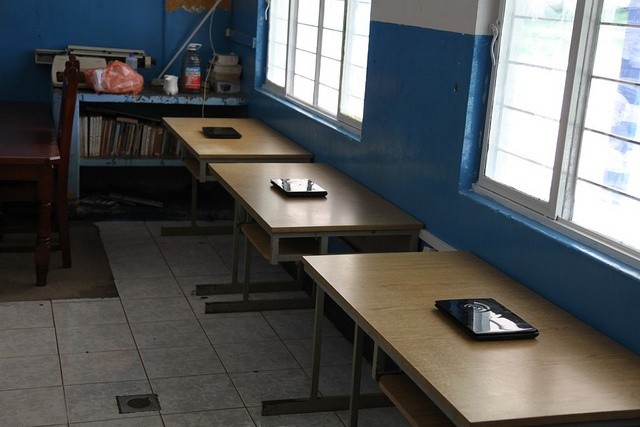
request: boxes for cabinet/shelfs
[53,93,246,225]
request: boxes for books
[79,114,180,157]
[209,53,242,82]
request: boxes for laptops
[202,126,241,139]
[433,297,539,341]
[270,177,328,198]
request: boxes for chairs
[3,56,73,271]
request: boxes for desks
[189,161,426,414]
[2,99,60,286]
[254,252,639,427]
[163,118,317,297]
